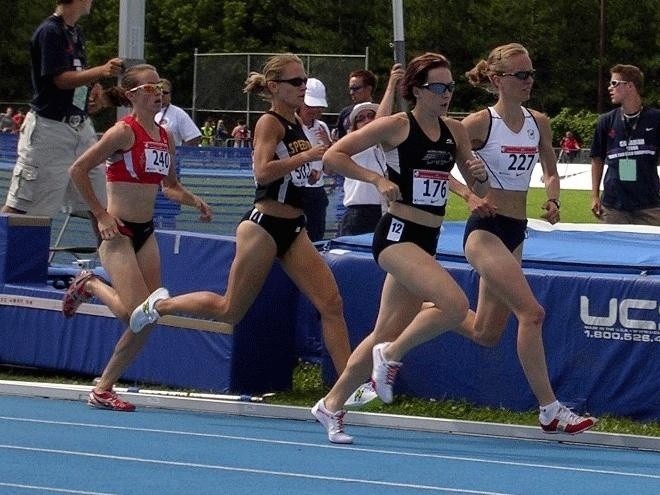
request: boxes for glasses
[497,69,536,79]
[130,84,162,92]
[419,81,455,94]
[268,77,307,86]
[356,112,375,121]
[349,85,366,91]
[610,80,627,87]
[163,90,170,95]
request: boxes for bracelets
[476,172,488,183]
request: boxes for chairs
[48,210,99,266]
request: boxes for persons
[62,63,212,411]
[560,131,581,163]
[0,1,122,256]
[1,107,15,135]
[201,121,213,146]
[590,64,660,227]
[311,53,490,443]
[217,120,228,148]
[238,125,251,148]
[231,119,245,148]
[297,77,333,242]
[130,53,378,405]
[420,43,597,436]
[341,64,405,238]
[335,70,377,239]
[153,79,202,230]
[14,108,25,132]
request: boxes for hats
[349,102,380,125]
[304,77,328,108]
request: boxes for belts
[55,111,85,127]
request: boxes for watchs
[547,199,561,210]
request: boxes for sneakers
[88,389,136,412]
[539,404,594,436]
[310,397,354,443]
[371,341,403,404]
[62,269,94,319]
[344,381,377,407]
[129,287,169,335]
